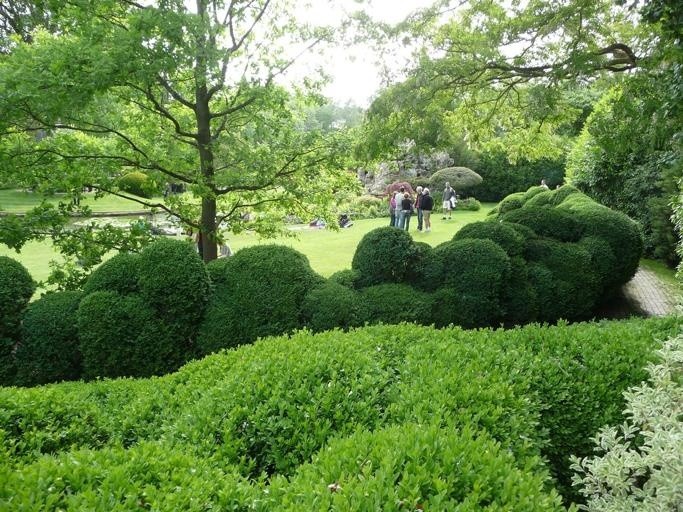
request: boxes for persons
[216,241,233,259]
[540,179,548,189]
[388,186,433,232]
[192,219,199,253]
[442,182,455,220]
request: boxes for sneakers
[443,217,451,219]
